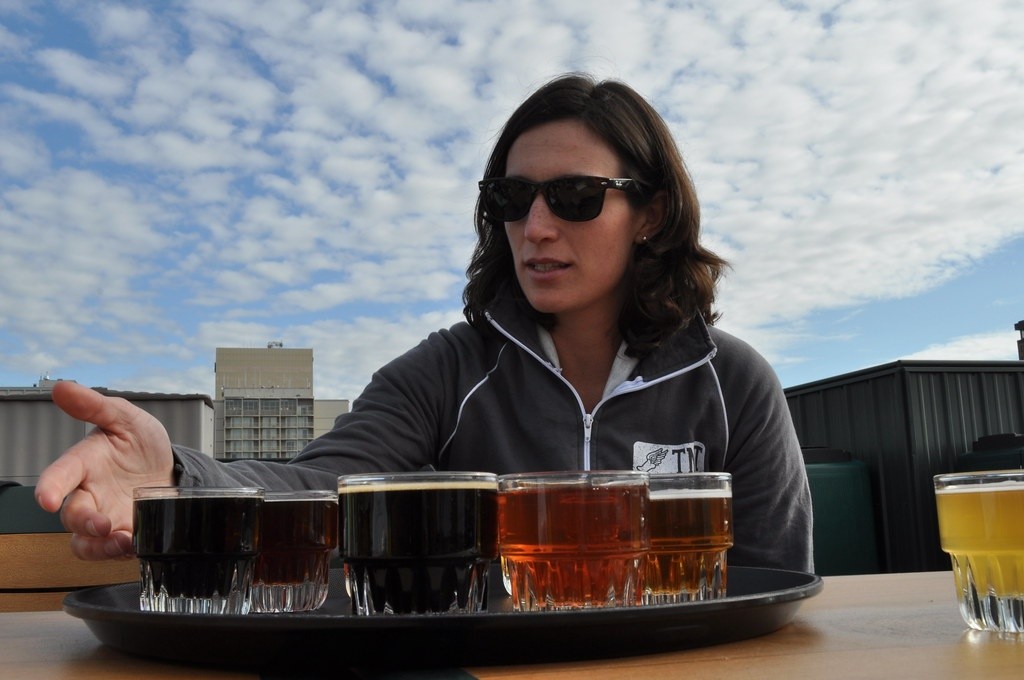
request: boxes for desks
[1,572,1024,680]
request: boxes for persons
[33,71,814,574]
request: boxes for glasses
[478,175,647,222]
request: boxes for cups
[649,473,735,606]
[933,468,1024,633]
[497,471,650,612]
[130,486,265,613]
[337,471,500,615]
[251,491,339,613]
